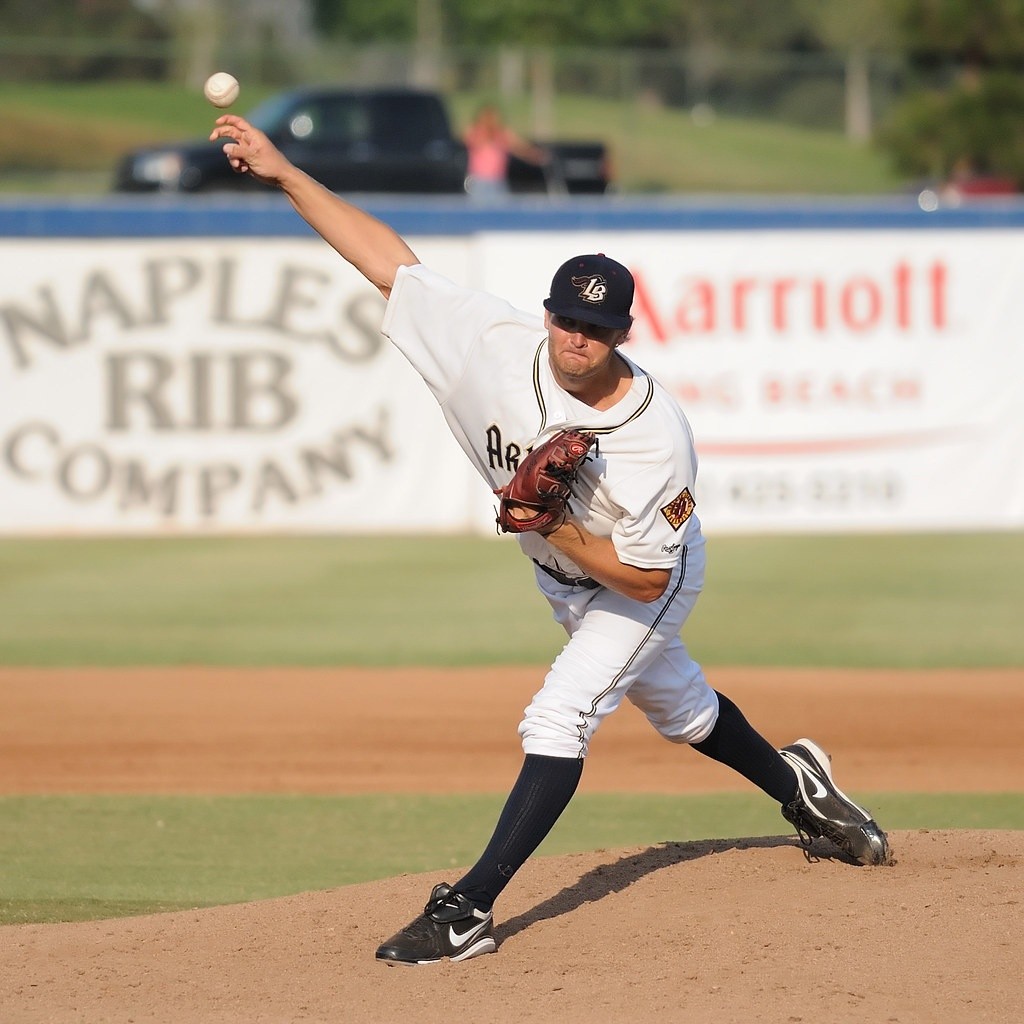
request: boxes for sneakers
[777,737,894,867]
[375,882,496,967]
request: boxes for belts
[532,558,601,590]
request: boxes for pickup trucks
[112,81,613,195]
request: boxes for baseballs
[201,72,242,103]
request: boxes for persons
[944,150,1019,205]
[453,108,552,192]
[209,115,888,969]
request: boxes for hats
[543,253,635,329]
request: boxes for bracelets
[539,510,567,540]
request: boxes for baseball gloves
[494,427,598,537]
